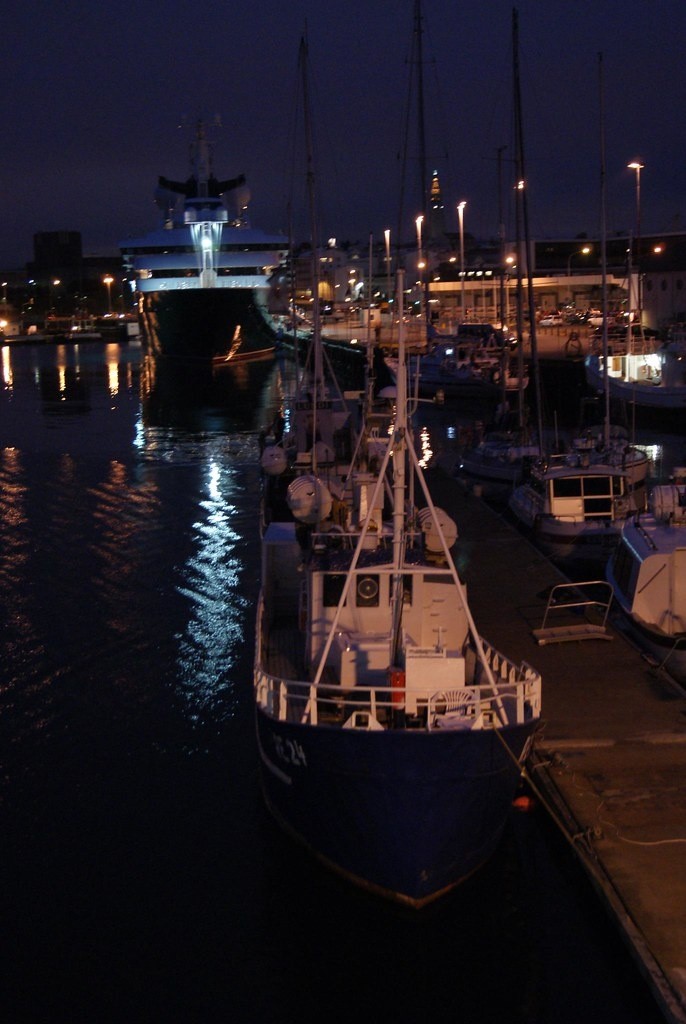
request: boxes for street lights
[2,273,116,317]
[382,159,663,305]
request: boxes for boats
[117,112,291,383]
[266,305,686,911]
